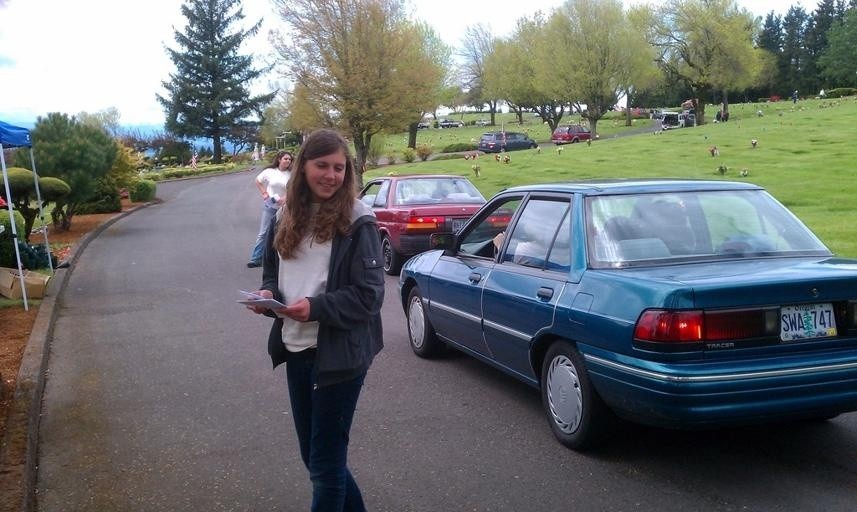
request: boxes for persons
[246,151,294,267]
[493,215,546,268]
[714,108,730,123]
[819,88,824,99]
[248,129,384,512]
[792,90,798,103]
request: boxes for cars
[351,175,513,275]
[438,119,459,129]
[766,94,781,102]
[614,106,700,130]
[551,124,600,145]
[473,118,491,125]
[418,117,431,129]
[395,177,855,448]
[264,146,278,152]
[533,109,542,116]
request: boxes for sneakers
[247,261,258,267]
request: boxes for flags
[192,147,199,170]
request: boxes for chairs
[614,238,670,262]
[407,194,430,203]
[447,193,470,203]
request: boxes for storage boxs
[0,267,51,299]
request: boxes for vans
[478,130,539,154]
